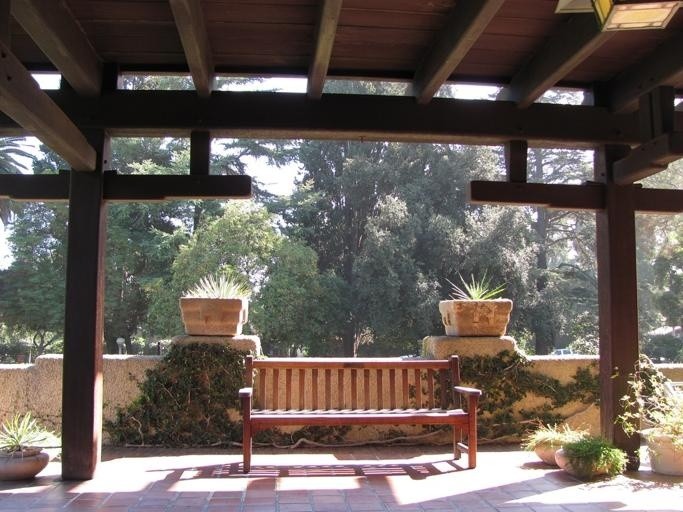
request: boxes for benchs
[238,354,482,474]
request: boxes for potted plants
[0,410,61,481]
[439,268,513,338]
[179,271,252,336]
[520,354,683,483]
